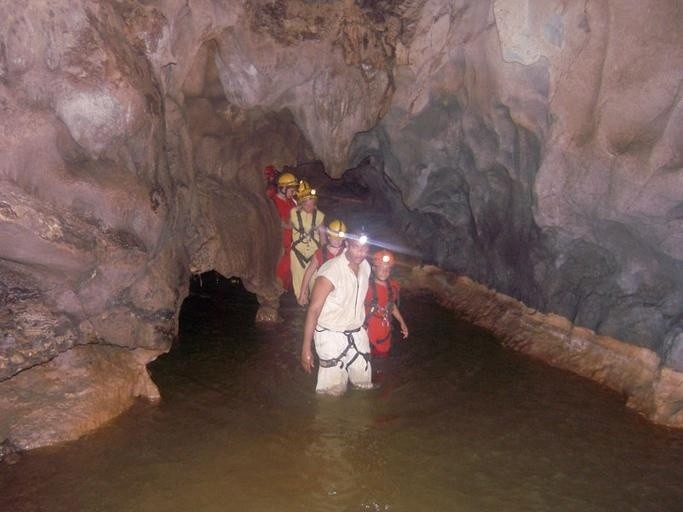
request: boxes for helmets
[296,179,320,202]
[348,228,371,246]
[326,219,348,240]
[371,249,396,266]
[277,172,299,187]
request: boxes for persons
[263,167,409,396]
